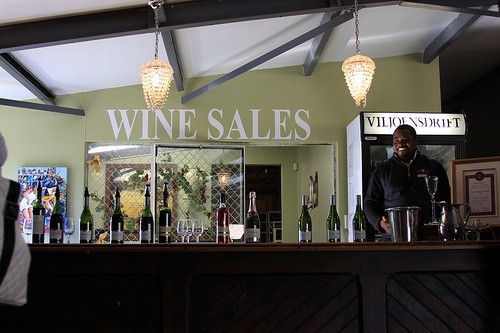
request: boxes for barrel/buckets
[385,206,420,242]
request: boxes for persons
[0,132,30,305]
[364,124,451,240]
[19,169,41,182]
[45,209,52,216]
[20,182,37,225]
[42,167,60,214]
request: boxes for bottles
[79,188,93,244]
[109,189,124,245]
[50,186,64,244]
[215,193,230,245]
[326,195,340,243]
[138,184,154,245]
[351,195,368,243]
[298,195,313,244]
[158,181,172,244]
[243,191,261,243]
[32,181,45,244]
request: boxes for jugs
[434,201,471,240]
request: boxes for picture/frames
[104,162,178,236]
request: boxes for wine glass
[177,219,204,244]
[63,217,75,244]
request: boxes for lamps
[216,165,232,193]
[340,0,376,108]
[138,0,174,112]
